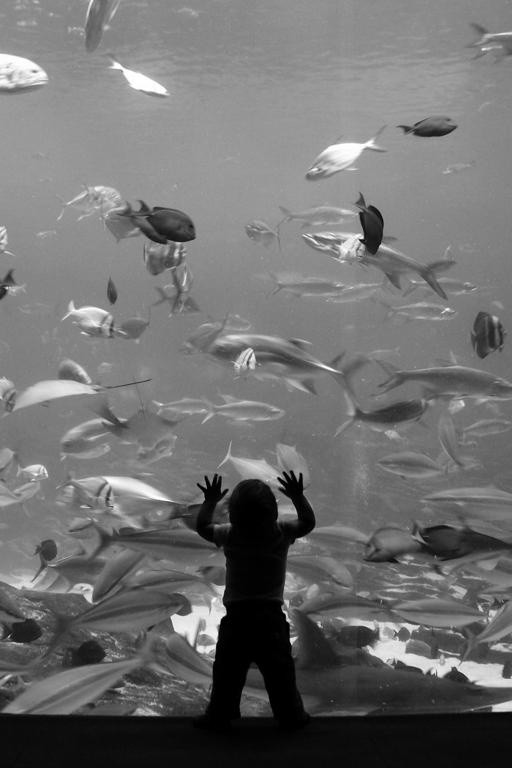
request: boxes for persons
[196,471,315,717]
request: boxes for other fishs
[0,183,510,717]
